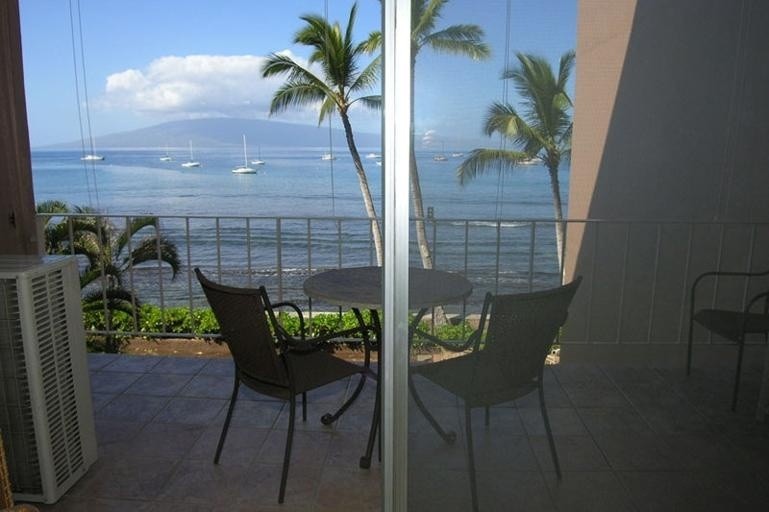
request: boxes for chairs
[191,266,382,503]
[686,266,769,411]
[402,274,586,510]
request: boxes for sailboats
[231,133,265,174]
[160,139,200,167]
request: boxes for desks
[302,267,474,472]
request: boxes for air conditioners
[0,254,101,504]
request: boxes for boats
[81,154,104,161]
[320,152,382,167]
[432,152,464,161]
[515,154,543,166]
